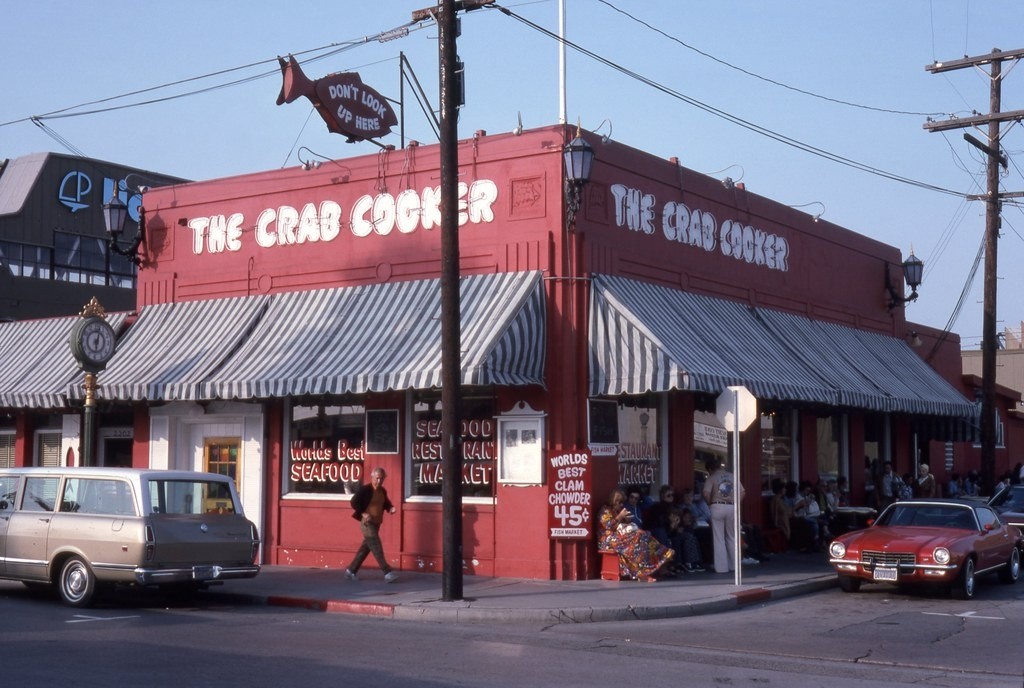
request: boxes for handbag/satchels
[617,521,638,535]
[692,519,710,530]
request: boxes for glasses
[667,494,673,497]
[619,500,622,504]
[629,495,640,500]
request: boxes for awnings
[57,272,546,392]
[0,312,126,408]
[589,273,979,418]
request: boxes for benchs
[598,549,623,581]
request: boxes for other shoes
[681,562,695,573]
[707,563,714,569]
[823,532,835,540]
[798,548,812,554]
[638,576,657,582]
[741,557,760,564]
[344,568,359,581]
[384,572,399,583]
[694,563,706,572]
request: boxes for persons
[703,459,746,578]
[595,485,759,582]
[773,460,1024,555]
[344,467,400,584]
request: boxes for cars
[0,467,262,607]
[827,498,1024,599]
[763,493,879,551]
[986,485,1024,535]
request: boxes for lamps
[298,146,351,177]
[562,111,595,236]
[100,179,146,269]
[512,110,523,137]
[589,117,613,145]
[704,162,744,191]
[786,201,826,223]
[883,241,923,311]
[910,330,923,347]
[136,184,148,195]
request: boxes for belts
[711,502,734,504]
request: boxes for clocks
[68,296,116,372]
